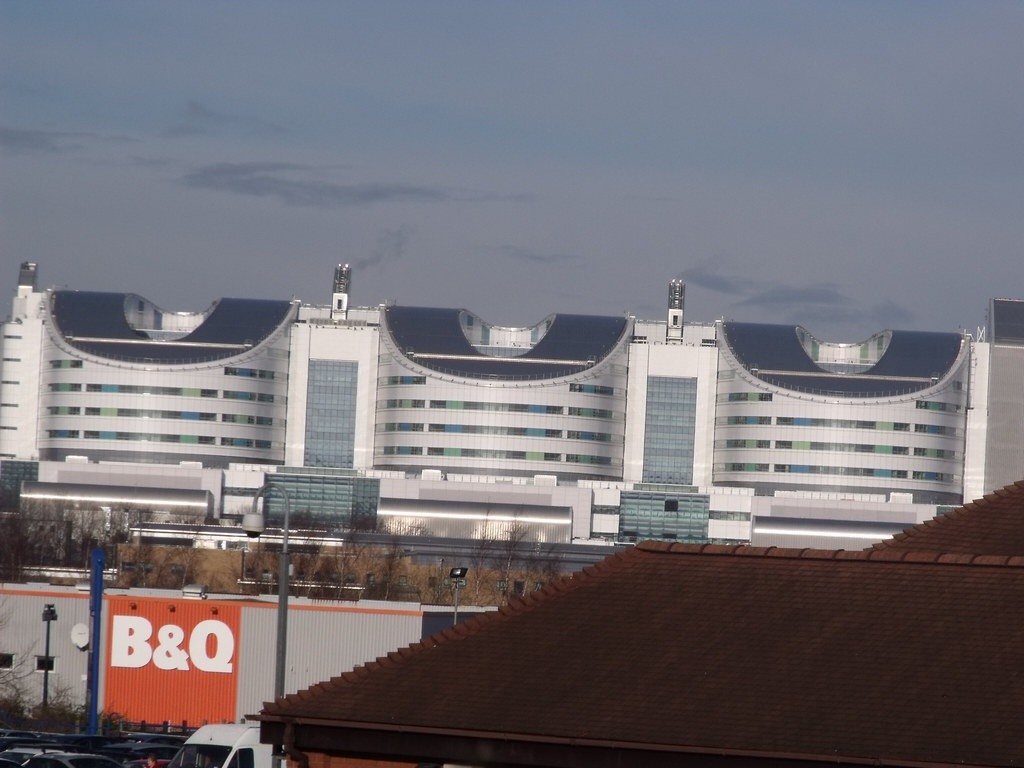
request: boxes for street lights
[450,567,468,629]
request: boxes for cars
[0,722,287,768]
